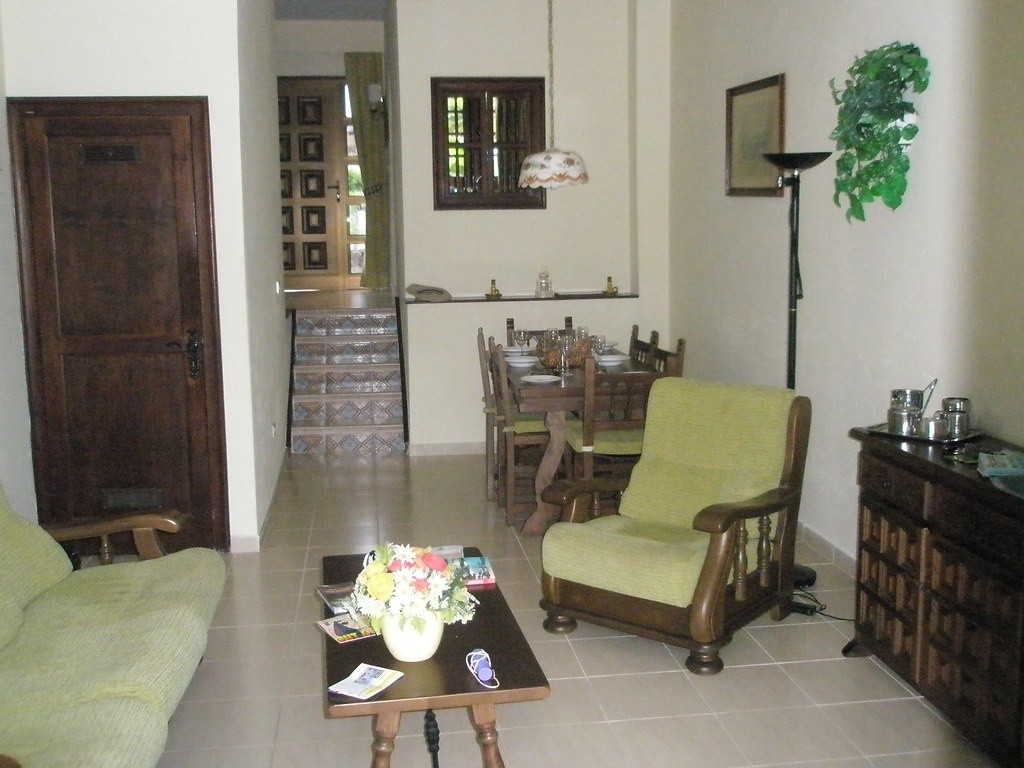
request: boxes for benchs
[0,483,227,768]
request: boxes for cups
[885,388,921,436]
[915,411,950,438]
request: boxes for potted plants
[830,41,931,226]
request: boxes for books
[316,613,381,644]
[444,556,496,586]
[990,476,1024,500]
[977,453,1024,476]
[315,582,355,614]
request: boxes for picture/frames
[724,72,786,198]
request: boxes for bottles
[536,270,553,299]
[945,397,970,438]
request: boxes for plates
[504,356,544,368]
[502,347,540,355]
[604,341,617,350]
[519,375,562,384]
[599,354,631,366]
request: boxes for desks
[486,347,660,537]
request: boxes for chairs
[541,376,813,676]
[477,317,687,526]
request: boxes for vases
[381,611,444,663]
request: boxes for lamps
[365,81,388,120]
[518,0,589,189]
[761,152,833,590]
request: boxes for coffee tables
[323,546,550,768]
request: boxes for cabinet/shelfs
[840,427,1024,768]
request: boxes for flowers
[350,541,480,628]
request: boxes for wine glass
[514,330,528,356]
[537,325,605,372]
